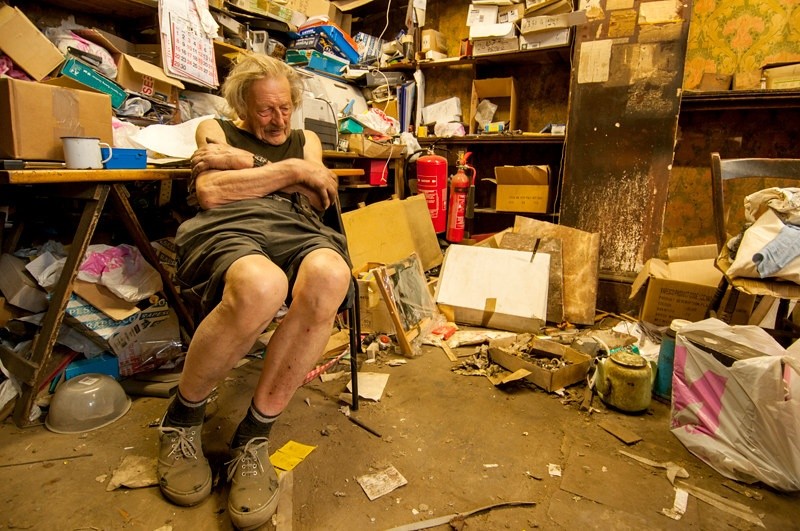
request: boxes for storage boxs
[1,1,796,393]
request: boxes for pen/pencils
[349,416,382,439]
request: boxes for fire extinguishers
[445,152,476,243]
[403,138,453,235]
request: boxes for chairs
[193,194,364,412]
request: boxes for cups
[60,134,112,170]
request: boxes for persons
[155,52,355,529]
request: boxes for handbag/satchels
[667,321,800,497]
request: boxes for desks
[0,169,365,429]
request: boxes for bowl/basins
[45,372,133,434]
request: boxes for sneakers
[225,429,280,530]
[156,404,213,509]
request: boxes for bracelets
[251,154,268,170]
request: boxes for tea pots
[595,347,658,415]
